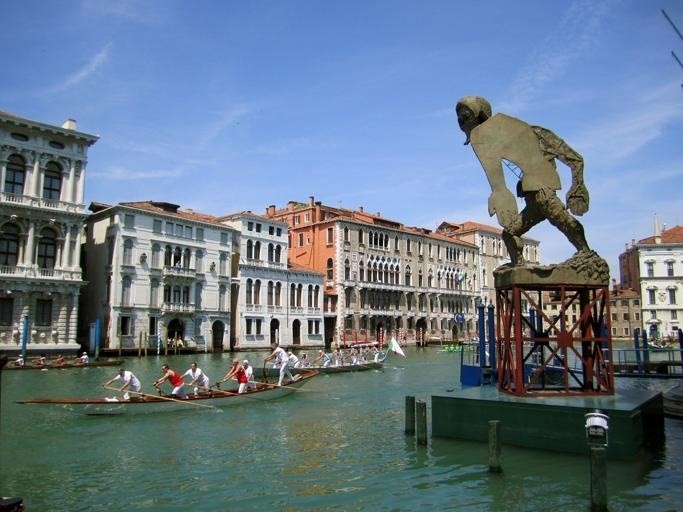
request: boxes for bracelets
[155,380,158,384]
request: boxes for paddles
[103,385,213,408]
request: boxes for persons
[167,335,183,348]
[75,352,89,365]
[273,344,380,368]
[31,352,46,365]
[456,95,607,270]
[152,364,187,399]
[222,357,249,394]
[52,353,65,366]
[241,360,257,389]
[101,367,143,400]
[8,354,24,368]
[263,342,295,387]
[179,362,215,398]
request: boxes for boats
[435,349,478,354]
[646,339,669,352]
[227,351,390,377]
[10,370,320,419]
[2,357,126,371]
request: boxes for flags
[388,338,406,360]
